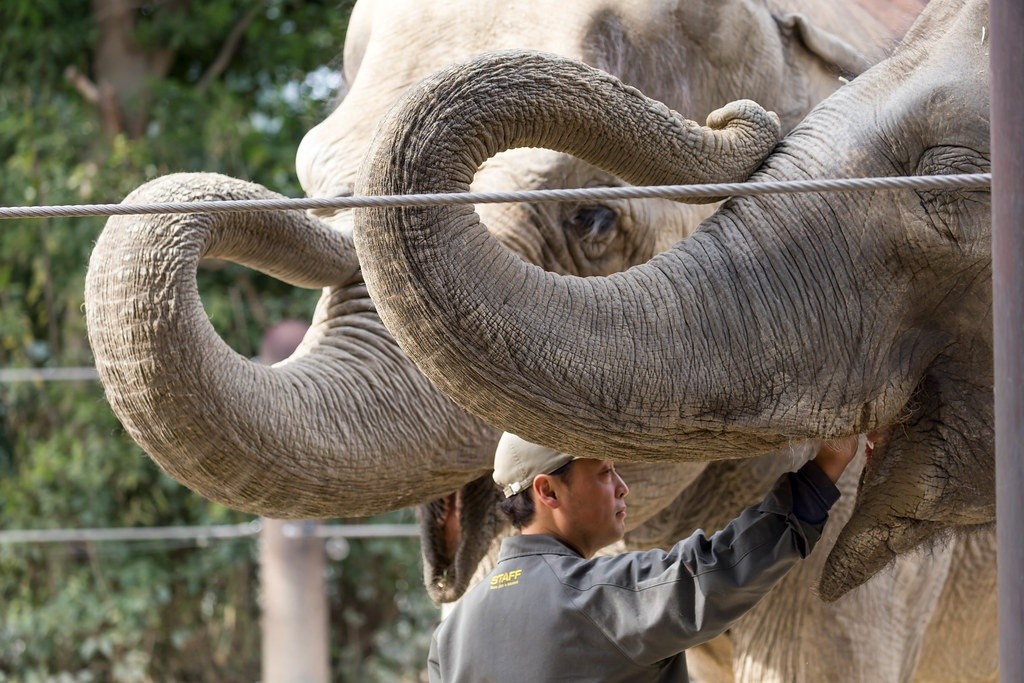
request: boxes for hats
[493,430,586,498]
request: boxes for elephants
[82,0,998,681]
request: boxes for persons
[425,430,860,683]
